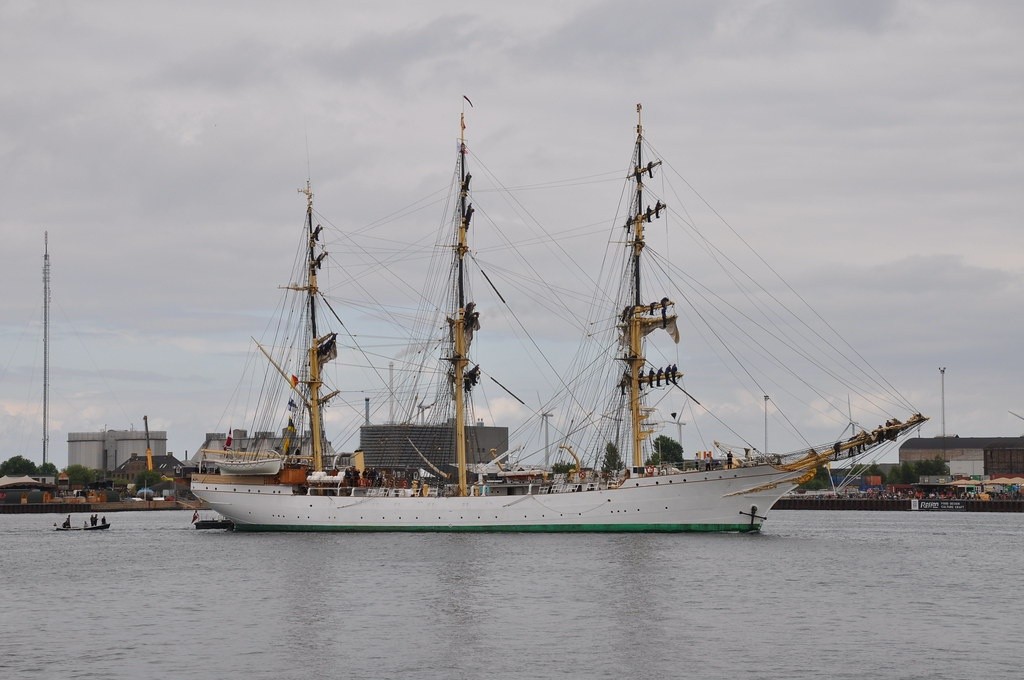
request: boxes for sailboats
[189,93,932,535]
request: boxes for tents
[0,475,38,489]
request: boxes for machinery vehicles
[143,415,153,471]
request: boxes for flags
[225,427,232,447]
[288,396,298,412]
[291,374,299,389]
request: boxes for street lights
[764,396,769,457]
[938,366,947,461]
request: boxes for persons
[62,514,106,529]
[695,453,699,471]
[704,454,711,471]
[344,466,381,487]
[789,488,883,500]
[883,487,1024,500]
[727,451,733,469]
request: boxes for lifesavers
[578,471,587,479]
[402,481,408,487]
[646,464,654,475]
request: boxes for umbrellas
[945,476,1024,500]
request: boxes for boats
[55,522,111,531]
